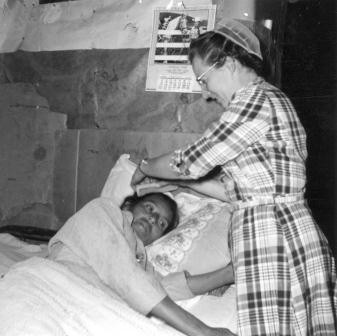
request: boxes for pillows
[97,154,235,294]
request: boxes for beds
[0,224,238,336]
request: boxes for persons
[131,18,337,336]
[45,190,239,335]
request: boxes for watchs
[138,157,148,176]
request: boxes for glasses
[195,61,217,86]
